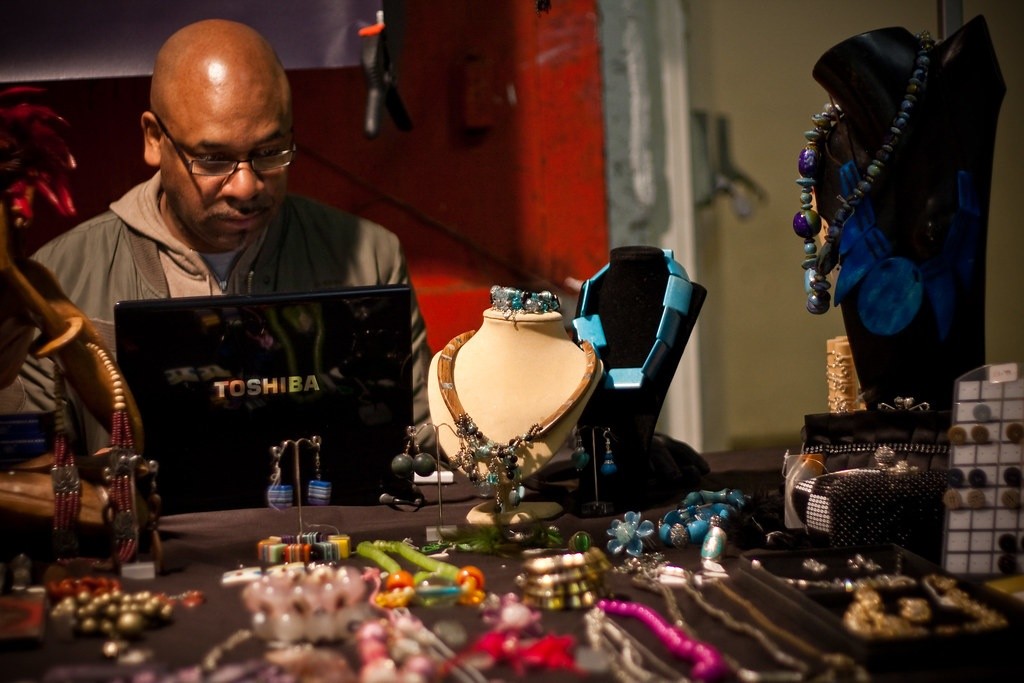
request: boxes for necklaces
[0,244,1023,682]
[793,17,990,342]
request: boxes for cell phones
[412,468,454,485]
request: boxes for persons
[1,17,431,497]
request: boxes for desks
[2,434,945,683]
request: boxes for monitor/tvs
[113,284,417,518]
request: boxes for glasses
[151,110,296,175]
